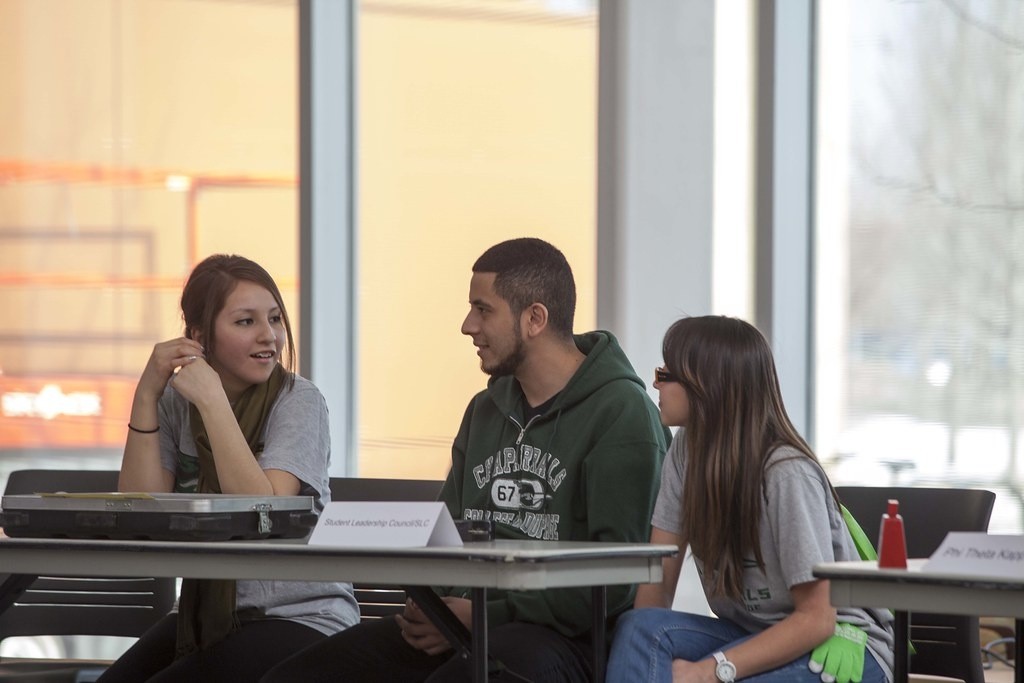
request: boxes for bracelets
[126,420,162,436]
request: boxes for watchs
[709,651,741,683]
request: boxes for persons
[255,236,680,682]
[598,313,915,682]
[94,252,366,683]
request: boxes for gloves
[807,622,868,683]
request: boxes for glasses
[655,366,678,384]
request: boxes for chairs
[1,467,174,681]
[825,485,996,681]
[310,474,452,632]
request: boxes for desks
[1,524,678,681]
[809,547,1024,682]
